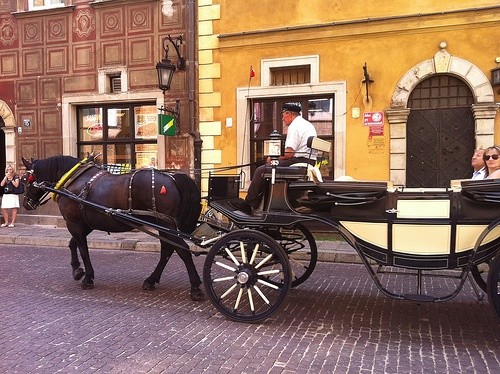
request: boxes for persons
[471,148,488,180]
[228,103,318,214]
[483,146,500,179]
[0,165,20,227]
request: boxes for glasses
[484,155,500,160]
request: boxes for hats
[280,104,301,113]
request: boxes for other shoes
[1,223,8,227]
[228,200,251,213]
[8,224,14,227]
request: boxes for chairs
[261,136,332,179]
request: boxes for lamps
[156,34,185,95]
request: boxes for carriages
[21,137,500,325]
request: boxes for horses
[21,156,204,302]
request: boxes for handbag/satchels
[8,182,25,194]
